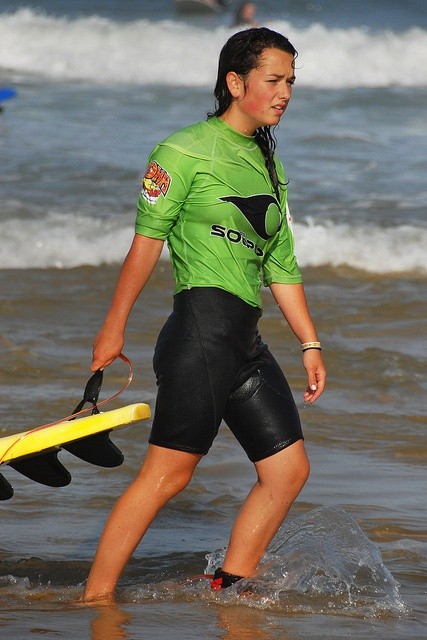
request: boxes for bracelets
[300,341,321,352]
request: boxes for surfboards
[0,352,151,500]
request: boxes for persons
[73,23,328,609]
[230,3,257,29]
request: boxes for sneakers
[210,568,271,601]
